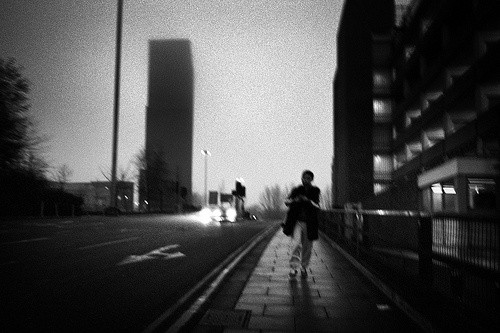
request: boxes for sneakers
[288,269,308,280]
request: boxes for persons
[282,170,324,277]
